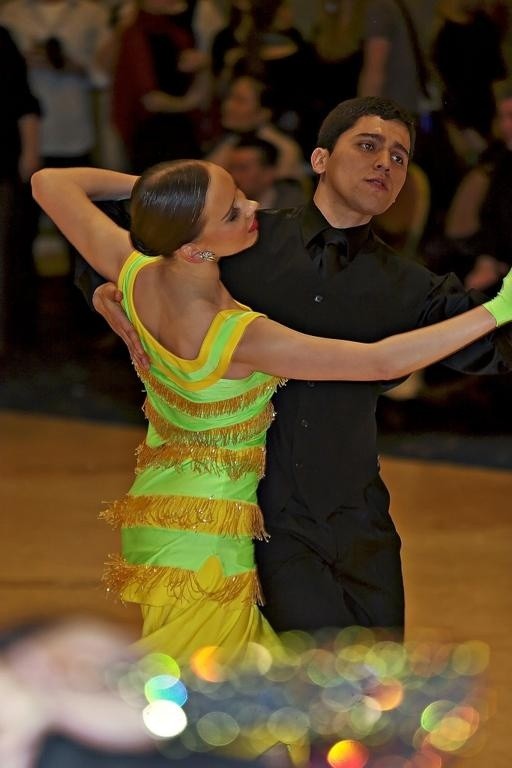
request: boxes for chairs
[373,160,431,259]
[269,172,312,211]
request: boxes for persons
[220,91,511,763]
[29,157,512,763]
[0,0,512,443]
